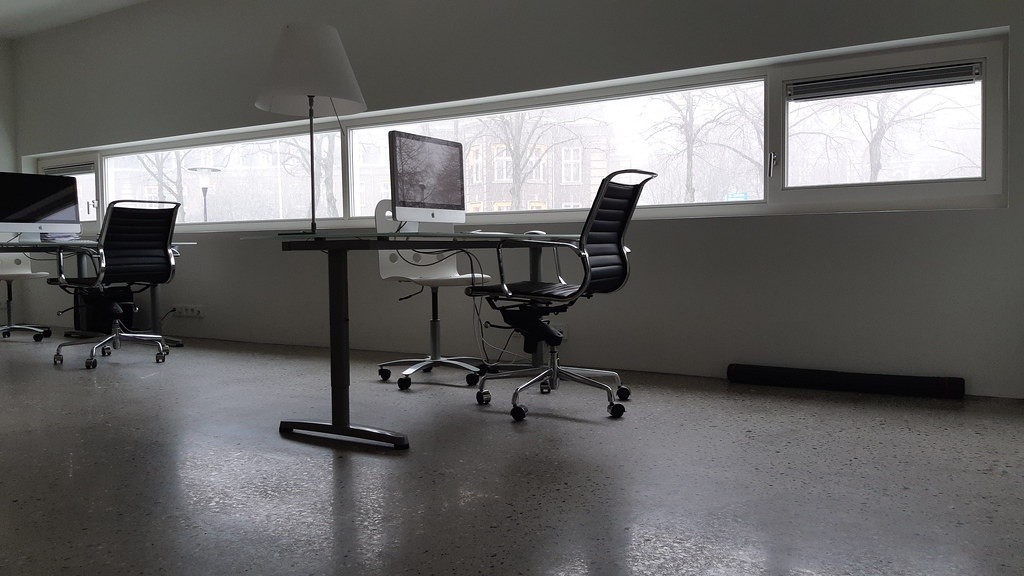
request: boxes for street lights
[186,164,222,223]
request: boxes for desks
[0,240,197,347]
[239,232,581,451]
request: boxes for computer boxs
[73,288,134,334]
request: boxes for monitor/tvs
[388,130,466,233]
[0,172,81,242]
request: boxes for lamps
[255,22,367,235]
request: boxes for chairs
[374,199,492,390]
[465,168,658,422]
[46,199,181,370]
[0,232,52,343]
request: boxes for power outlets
[511,323,568,340]
[169,304,204,319]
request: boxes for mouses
[524,230,546,235]
